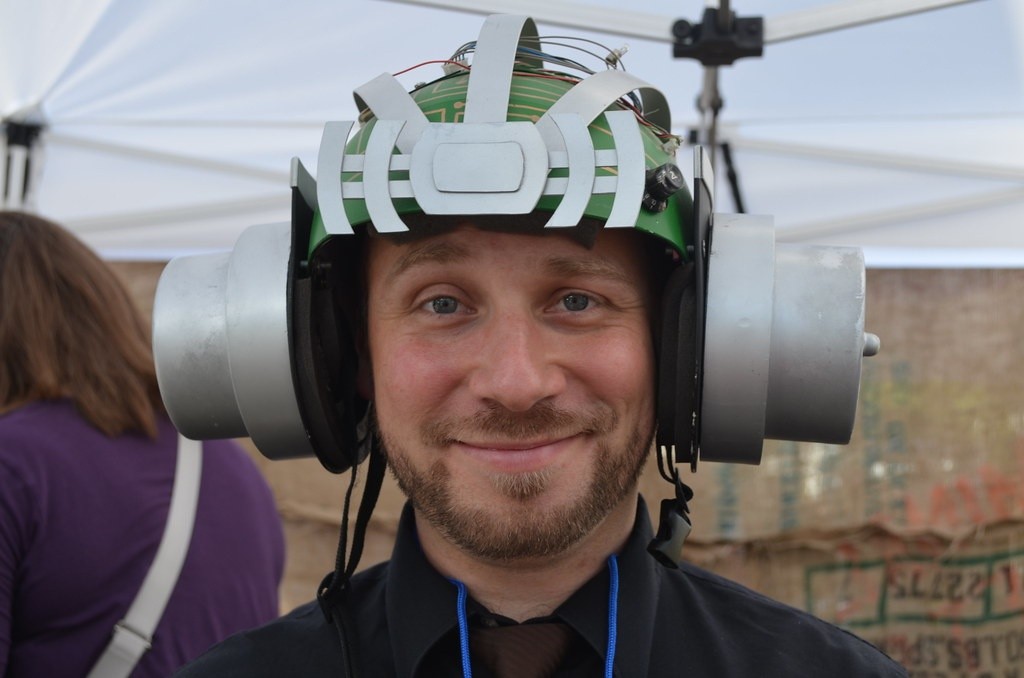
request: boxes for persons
[0,211,275,678]
[153,16,917,678]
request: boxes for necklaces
[452,554,619,678]
